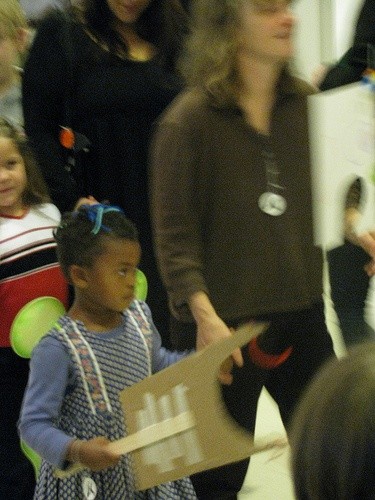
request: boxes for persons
[17,195,234,500]
[0,0,375,500]
[287,339,375,500]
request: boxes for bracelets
[70,438,87,465]
